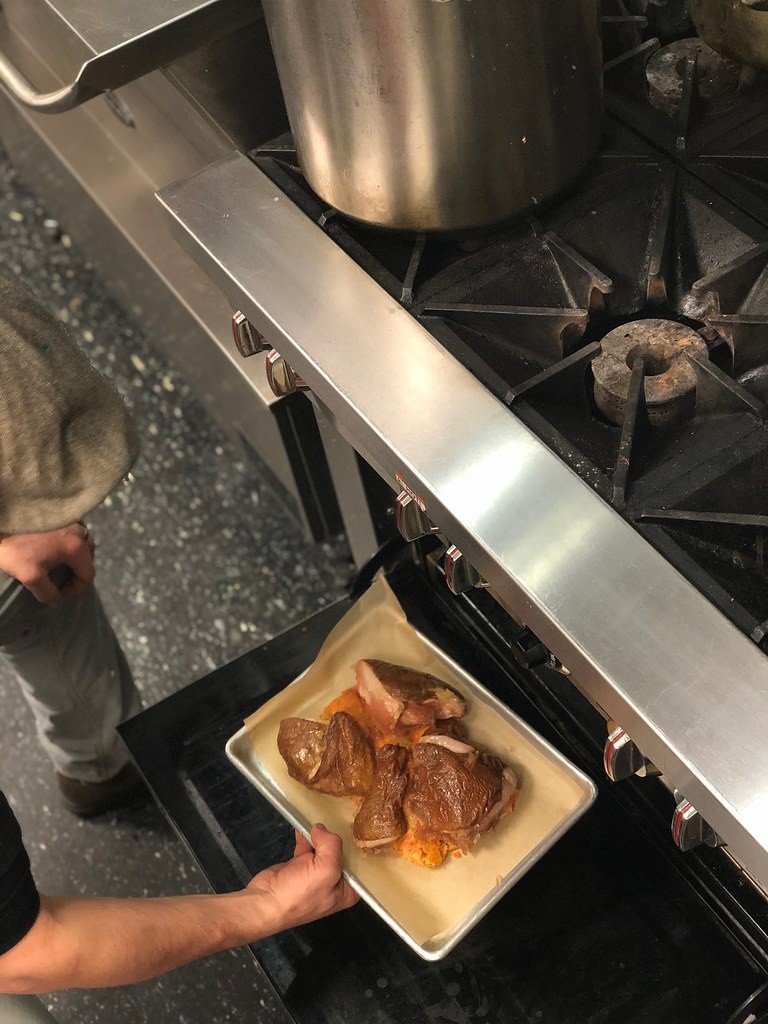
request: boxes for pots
[247,1,767,651]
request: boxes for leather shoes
[56,761,145,820]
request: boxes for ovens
[113,150,768,1024]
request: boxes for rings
[83,525,90,540]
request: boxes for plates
[225,605,598,962]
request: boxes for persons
[0,253,167,817]
[0,785,361,1024]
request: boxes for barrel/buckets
[261,1,604,231]
